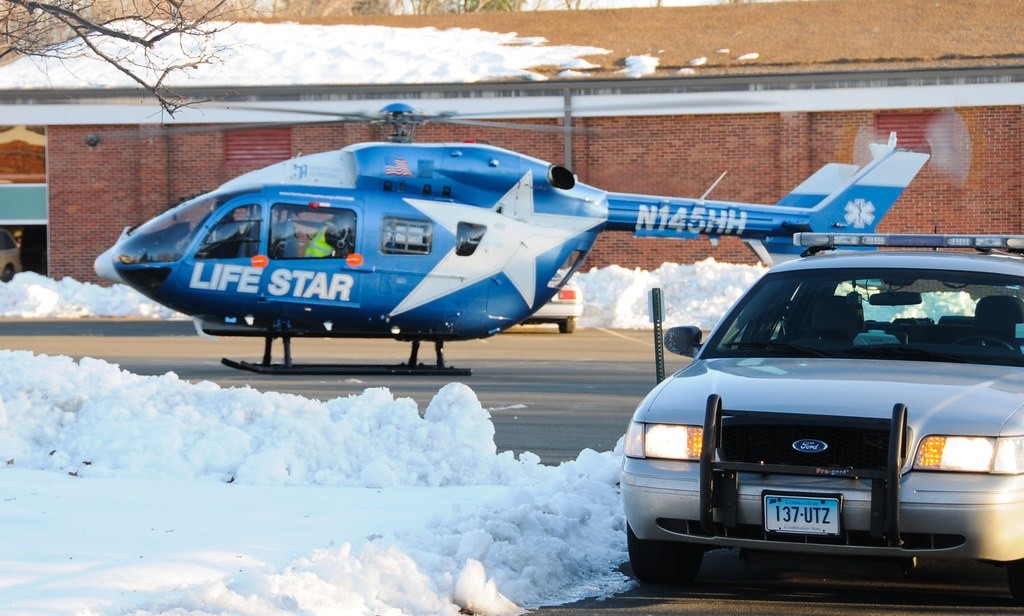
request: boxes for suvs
[620,232,1024,601]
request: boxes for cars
[0,226,23,282]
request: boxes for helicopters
[93,102,931,377]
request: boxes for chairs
[975,294,1024,350]
[791,295,864,350]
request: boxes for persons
[306,211,354,259]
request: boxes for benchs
[863,322,975,345]
